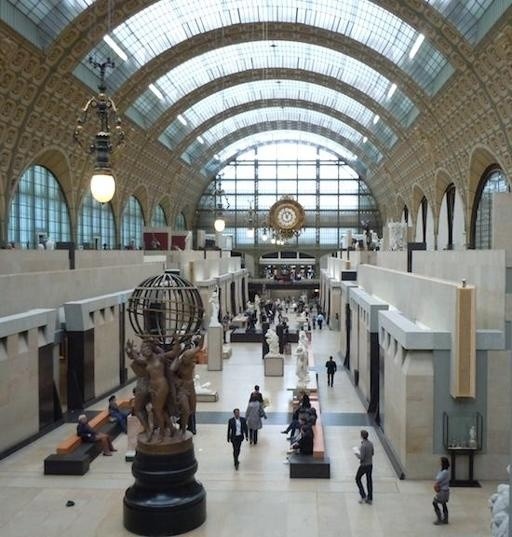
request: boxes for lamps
[72,2,133,208]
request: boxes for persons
[221,290,331,334]
[76,412,117,456]
[105,396,128,435]
[432,455,452,527]
[352,428,375,506]
[325,354,338,388]
[225,408,248,471]
[245,382,320,466]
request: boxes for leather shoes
[358,495,373,505]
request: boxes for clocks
[274,204,300,230]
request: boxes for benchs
[44,398,132,476]
[288,396,331,479]
